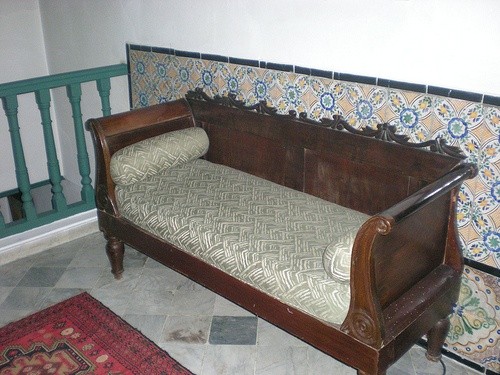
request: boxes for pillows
[323,222,365,284]
[110,126,210,185]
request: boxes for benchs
[84,94,479,375]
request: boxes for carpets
[0,293,197,375]
